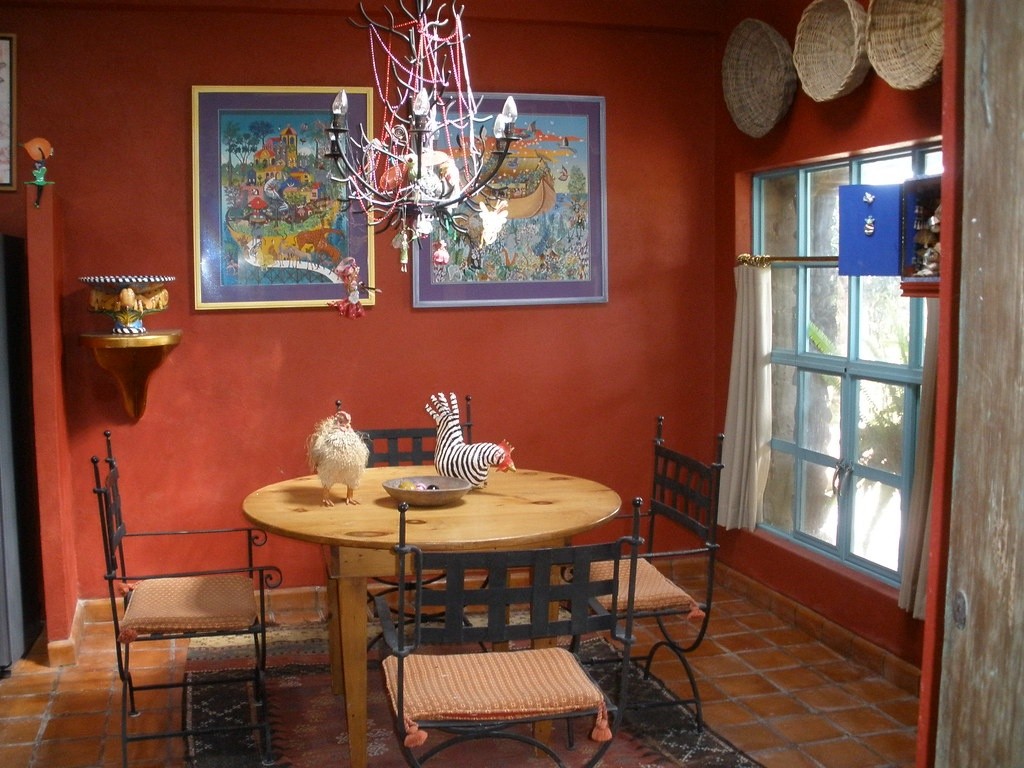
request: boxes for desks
[243,465,624,768]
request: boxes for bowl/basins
[78,275,176,295]
[382,475,472,508]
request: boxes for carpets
[183,603,766,768]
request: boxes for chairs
[560,411,725,750]
[89,428,284,768]
[371,496,646,768]
[335,395,492,653]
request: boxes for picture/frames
[0,32,18,193]
[405,92,609,308]
[190,86,375,310]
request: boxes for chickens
[426,392,516,489]
[307,411,368,506]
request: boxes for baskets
[791,1,870,104]
[719,19,797,140]
[864,1,946,90]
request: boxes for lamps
[324,0,519,273]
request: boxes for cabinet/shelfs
[838,176,939,297]
